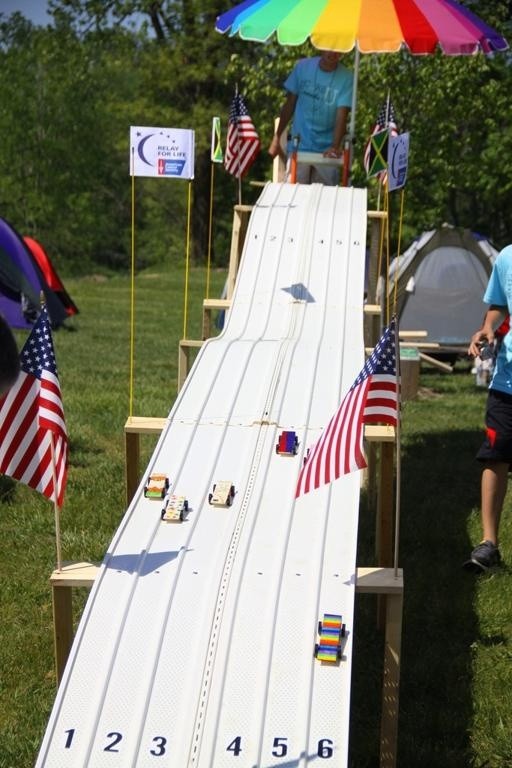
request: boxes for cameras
[477,338,498,360]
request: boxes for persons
[1,317,22,406]
[453,240,511,583]
[266,48,355,186]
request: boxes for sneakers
[461,540,500,574]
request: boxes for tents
[375,218,508,355]
[0,216,72,332]
[23,235,81,316]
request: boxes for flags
[222,91,261,182]
[211,116,223,164]
[368,129,390,178]
[1,301,72,509]
[387,132,407,193]
[294,314,402,502]
[363,95,399,189]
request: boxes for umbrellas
[210,0,509,189]
